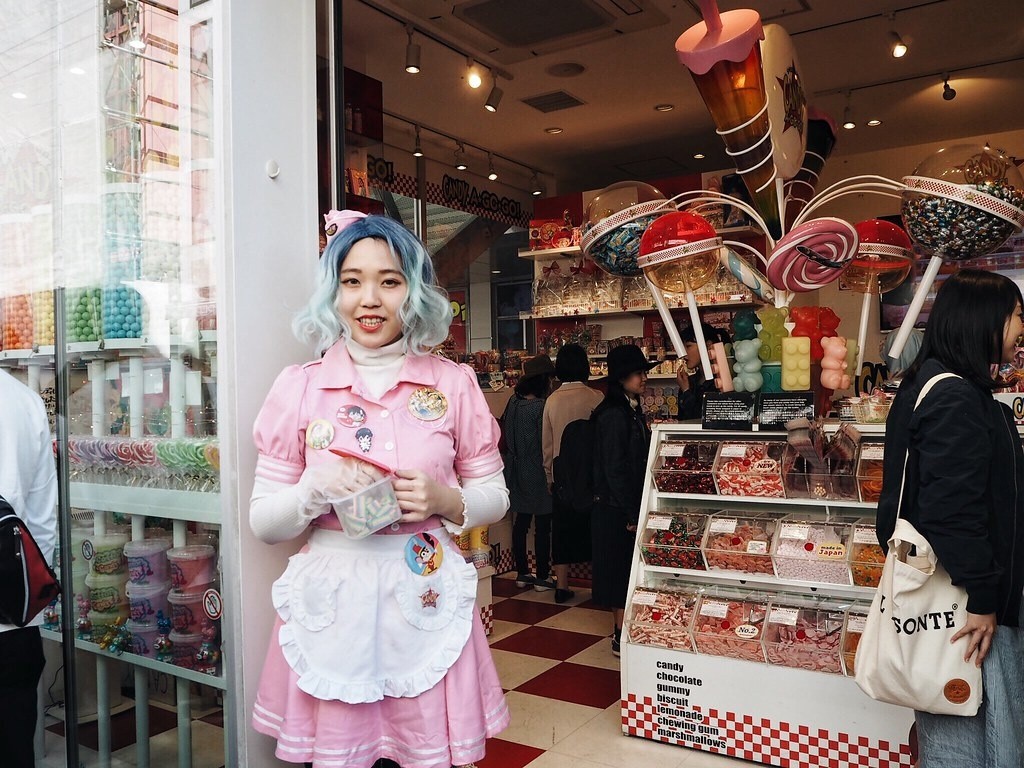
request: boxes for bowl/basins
[851,405,889,423]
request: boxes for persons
[876,268,1024,768]
[0,368,59,768]
[248,210,510,768]
[500,322,737,656]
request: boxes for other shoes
[555,589,575,603]
[515,575,537,588]
[611,634,620,656]
[534,578,556,592]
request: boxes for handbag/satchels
[495,394,516,454]
[854,518,983,716]
[0,494,61,627]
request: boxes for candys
[724,303,851,392]
[52,437,220,472]
[712,243,774,304]
[0,171,216,350]
[583,214,660,274]
[341,492,400,536]
[902,180,1023,261]
[766,217,912,295]
[638,211,724,293]
[632,444,885,673]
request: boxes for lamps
[842,89,856,129]
[453,139,468,171]
[405,27,423,75]
[413,126,422,156]
[884,14,908,58]
[488,152,498,181]
[864,89,883,126]
[527,169,542,196]
[941,72,957,101]
[482,70,504,111]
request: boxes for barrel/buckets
[56,519,216,668]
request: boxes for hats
[599,345,663,383]
[523,354,556,376]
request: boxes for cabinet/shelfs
[620,423,920,768]
[517,227,770,382]
[0,330,227,692]
[317,54,384,148]
